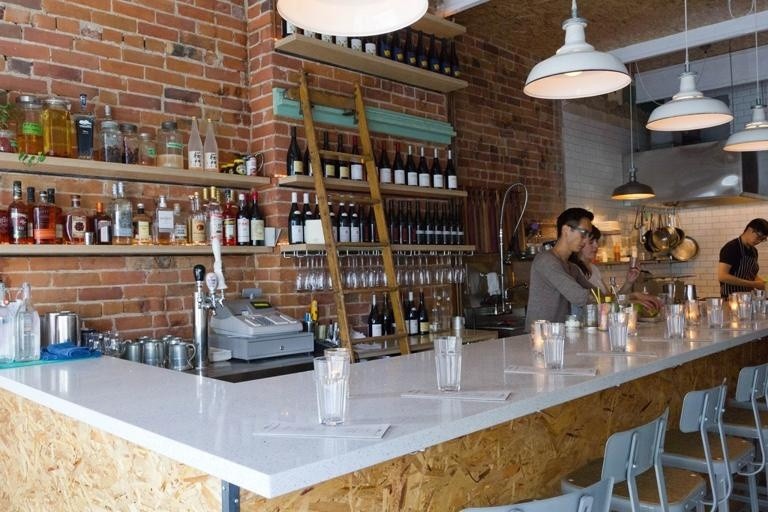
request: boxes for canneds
[233,159,244,174]
[220,164,226,173]
[244,156,258,176]
[226,163,234,173]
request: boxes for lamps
[277,0,430,39]
[724,104,763,152]
[522,18,634,99]
[645,72,734,132]
[610,180,656,202]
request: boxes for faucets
[504,281,529,315]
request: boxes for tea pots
[100,331,132,359]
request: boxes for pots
[674,227,685,245]
[653,214,668,251]
[660,215,677,246]
[643,213,659,254]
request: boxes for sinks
[465,312,526,332]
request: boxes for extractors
[626,138,767,210]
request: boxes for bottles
[236,193,250,246]
[396,200,408,245]
[119,122,139,164]
[157,121,184,169]
[320,34,334,44]
[97,118,124,162]
[302,192,314,244]
[444,149,458,190]
[417,30,429,71]
[334,36,350,48]
[204,119,220,173]
[382,198,388,226]
[379,141,393,184]
[387,199,398,244]
[108,183,118,218]
[364,37,378,55]
[350,37,364,53]
[314,194,321,219]
[417,146,431,188]
[138,132,157,166]
[449,41,460,79]
[406,200,417,245]
[406,291,419,336]
[613,241,621,262]
[187,116,204,171]
[378,34,392,60]
[250,190,265,247]
[428,34,441,74]
[287,192,304,245]
[367,294,383,337]
[223,190,237,246]
[371,139,379,182]
[448,202,456,245]
[173,202,188,246]
[111,181,133,245]
[358,203,370,243]
[187,194,195,217]
[281,19,299,39]
[32,191,57,244]
[391,31,405,63]
[406,145,418,186]
[432,147,444,189]
[382,292,394,336]
[304,145,314,176]
[348,195,360,243]
[368,204,379,242]
[14,95,42,156]
[63,194,91,245]
[42,96,72,157]
[336,194,351,243]
[71,93,96,160]
[424,200,435,245]
[337,134,350,180]
[350,135,367,181]
[440,202,451,245]
[133,203,154,246]
[301,28,320,40]
[91,201,113,245]
[153,194,175,245]
[189,192,206,244]
[328,194,338,242]
[321,131,337,178]
[455,204,464,245]
[405,28,417,67]
[47,188,63,245]
[286,126,304,176]
[439,38,452,76]
[230,189,240,215]
[201,187,210,214]
[432,202,442,245]
[25,186,37,245]
[206,186,223,246]
[417,287,429,335]
[8,180,28,244]
[414,200,424,245]
[393,143,406,185]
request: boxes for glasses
[568,224,592,237]
[755,232,767,242]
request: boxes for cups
[168,342,196,371]
[313,356,351,425]
[540,323,565,370]
[169,337,182,343]
[142,339,168,368]
[624,307,637,336]
[686,300,699,321]
[736,292,752,323]
[531,320,550,351]
[607,312,629,354]
[756,300,768,319]
[160,334,173,342]
[293,253,466,291]
[700,301,707,320]
[752,290,765,313]
[706,298,723,329]
[324,348,351,373]
[127,342,143,364]
[433,335,462,392]
[664,304,685,344]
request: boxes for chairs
[459,476,617,512]
[596,407,683,512]
[704,365,764,512]
[560,383,733,512]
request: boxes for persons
[521,209,662,336]
[568,225,643,317]
[718,218,768,301]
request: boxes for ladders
[297,66,411,365]
